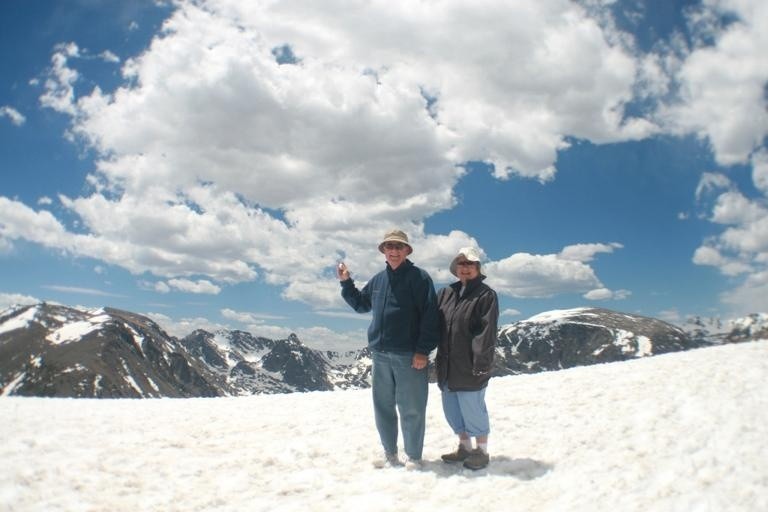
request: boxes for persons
[435,247,499,469]
[336,228,442,471]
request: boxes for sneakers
[374,454,398,467]
[441,444,469,462]
[463,448,489,468]
[406,458,422,470]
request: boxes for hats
[450,247,485,278]
[378,230,412,255]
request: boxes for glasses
[458,261,472,265]
[384,244,403,249]
